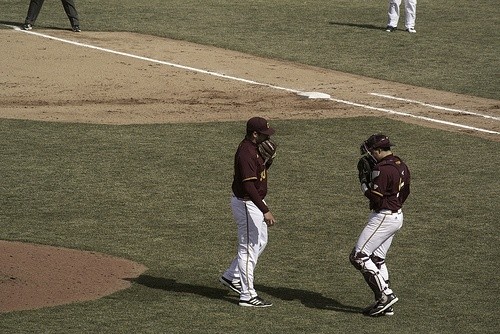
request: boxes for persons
[20,0,81,32]
[220,117,278,308]
[349,134,410,316]
[385,0,417,33]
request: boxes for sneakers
[239,296,273,307]
[386,26,393,32]
[21,23,32,29]
[219,274,243,294]
[407,28,416,33]
[369,290,399,315]
[363,306,394,315]
[72,26,81,32]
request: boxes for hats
[367,135,396,150]
[247,117,275,135]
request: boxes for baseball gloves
[356,155,374,180]
[257,139,278,161]
